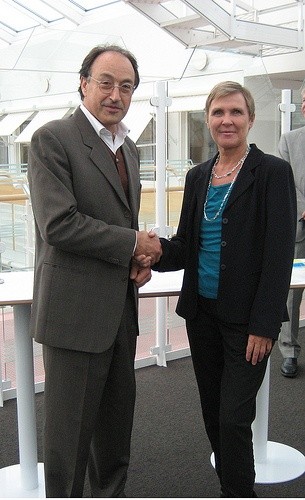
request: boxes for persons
[133,81,298,498]
[274,83,305,378]
[27,45,161,498]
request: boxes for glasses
[89,75,135,97]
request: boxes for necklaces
[205,144,250,222]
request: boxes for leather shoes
[282,358,299,378]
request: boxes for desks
[0,258,305,498]
